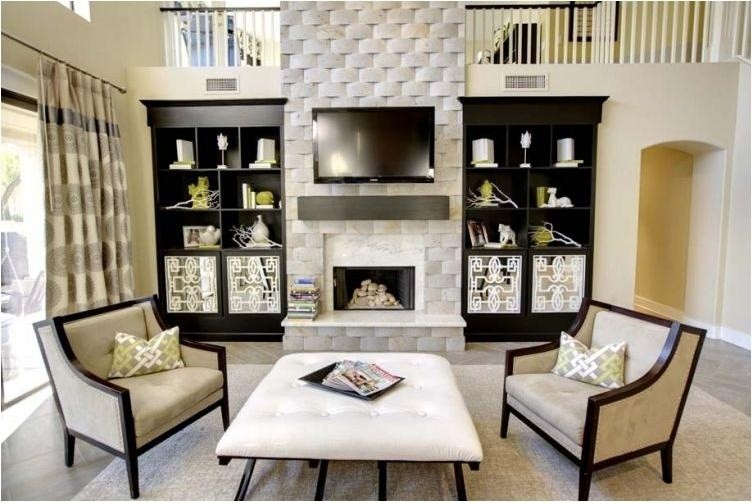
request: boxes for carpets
[71,363,751,501]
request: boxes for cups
[537,187,550,207]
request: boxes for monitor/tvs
[311,107,436,184]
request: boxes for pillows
[107,325,186,379]
[549,331,627,389]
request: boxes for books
[322,360,400,396]
[286,278,322,323]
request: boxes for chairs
[32,294,231,499]
[500,297,708,501]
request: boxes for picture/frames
[568,1,619,42]
[182,225,207,248]
[468,220,489,247]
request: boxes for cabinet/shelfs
[489,23,541,64]
[457,96,610,342]
[139,98,288,343]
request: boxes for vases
[251,214,270,243]
[480,179,494,201]
